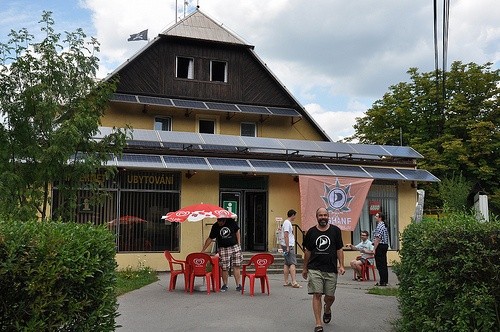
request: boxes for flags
[127,29,148,41]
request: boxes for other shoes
[374,282,389,286]
[221,284,228,292]
[353,276,364,281]
[236,284,242,291]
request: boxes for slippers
[292,282,300,288]
[283,282,291,286]
[323,303,332,324]
[315,326,323,332]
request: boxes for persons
[201,217,246,292]
[373,212,389,286]
[282,209,302,288]
[302,207,346,332]
[346,230,375,282]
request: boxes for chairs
[164,249,215,295]
[241,253,275,298]
[354,255,376,281]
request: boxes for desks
[213,256,223,292]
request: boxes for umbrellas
[107,215,148,226]
[161,202,238,247]
